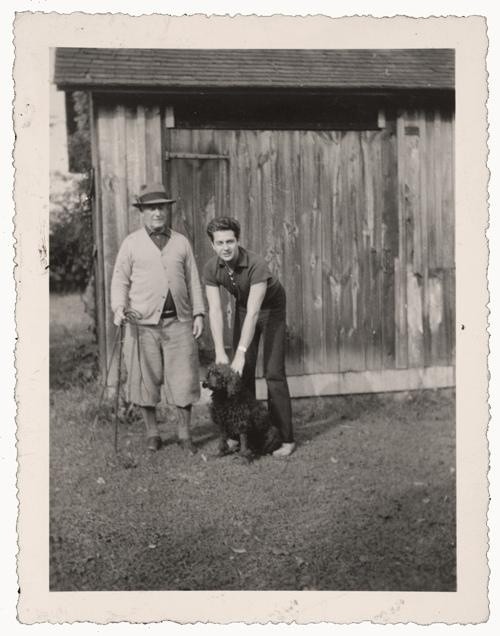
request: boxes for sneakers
[179,438,198,457]
[224,437,240,454]
[272,441,296,458]
[147,435,162,451]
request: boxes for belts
[160,311,177,318]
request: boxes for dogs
[202,362,282,467]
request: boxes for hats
[132,182,176,208]
